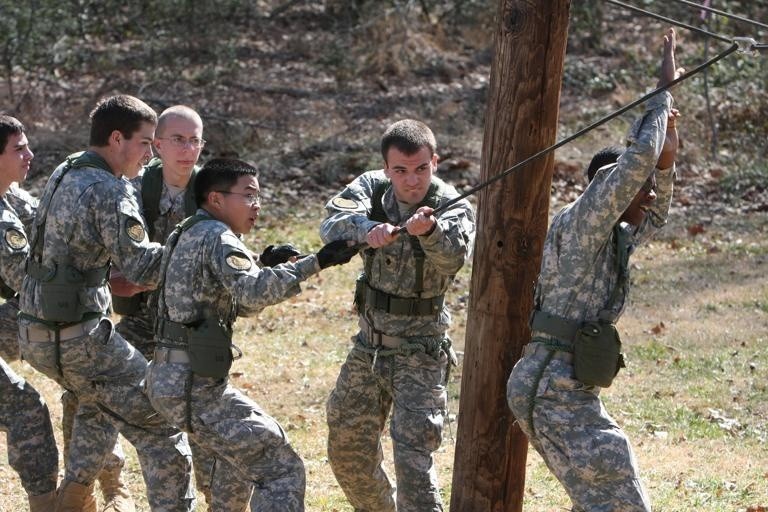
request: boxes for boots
[27,490,57,512]
[54,479,89,512]
[82,483,97,512]
[198,487,212,512]
[96,466,136,512]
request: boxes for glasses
[643,180,658,193]
[158,136,207,148]
[216,190,263,204]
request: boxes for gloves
[260,244,300,267]
[316,240,361,270]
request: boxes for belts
[356,318,408,351]
[16,318,103,344]
[148,348,188,364]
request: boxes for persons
[49,105,215,511]
[505,28,680,512]
[145,160,306,512]
[0,116,58,511]
[17,96,194,510]
[320,120,475,511]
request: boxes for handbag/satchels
[39,265,88,326]
[572,322,624,391]
[189,316,233,381]
